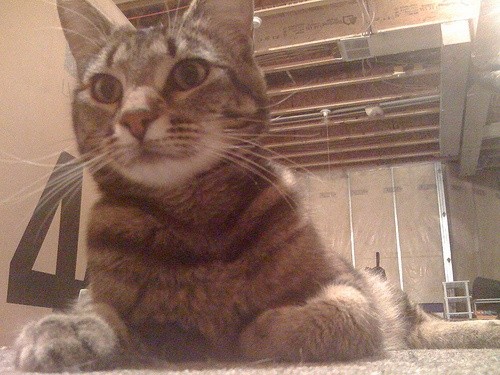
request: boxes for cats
[11,0,500,373]
